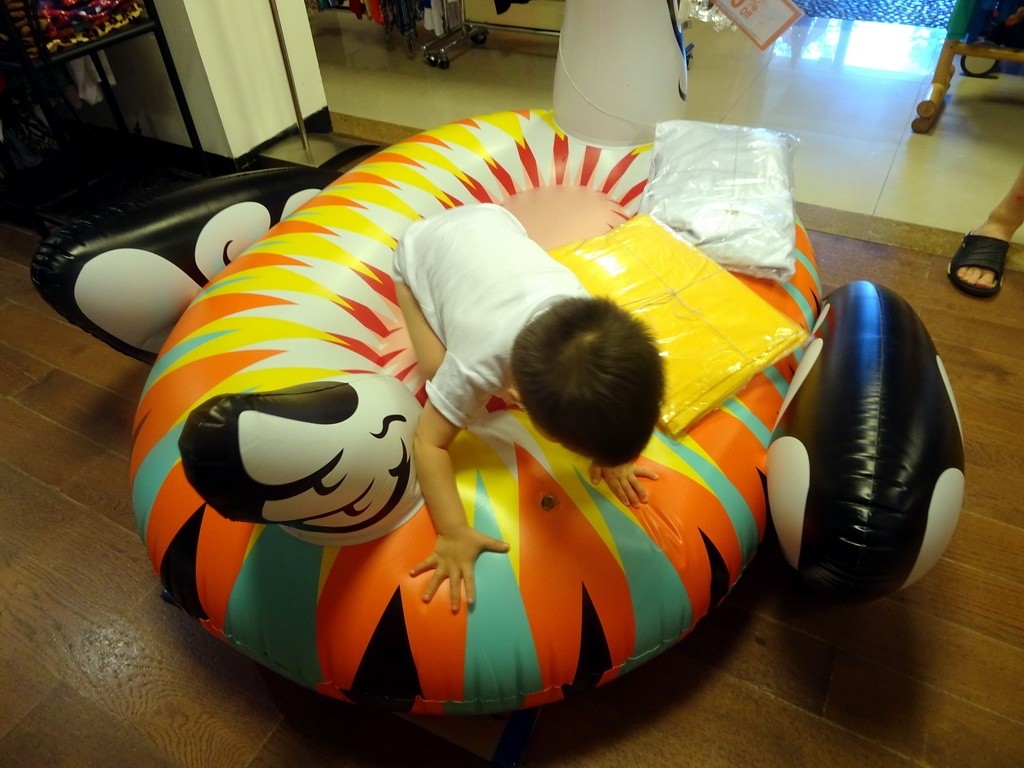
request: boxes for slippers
[947,229,1010,297]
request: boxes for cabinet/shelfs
[0,0,209,238]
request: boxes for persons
[392,203,666,612]
[947,167,1024,297]
